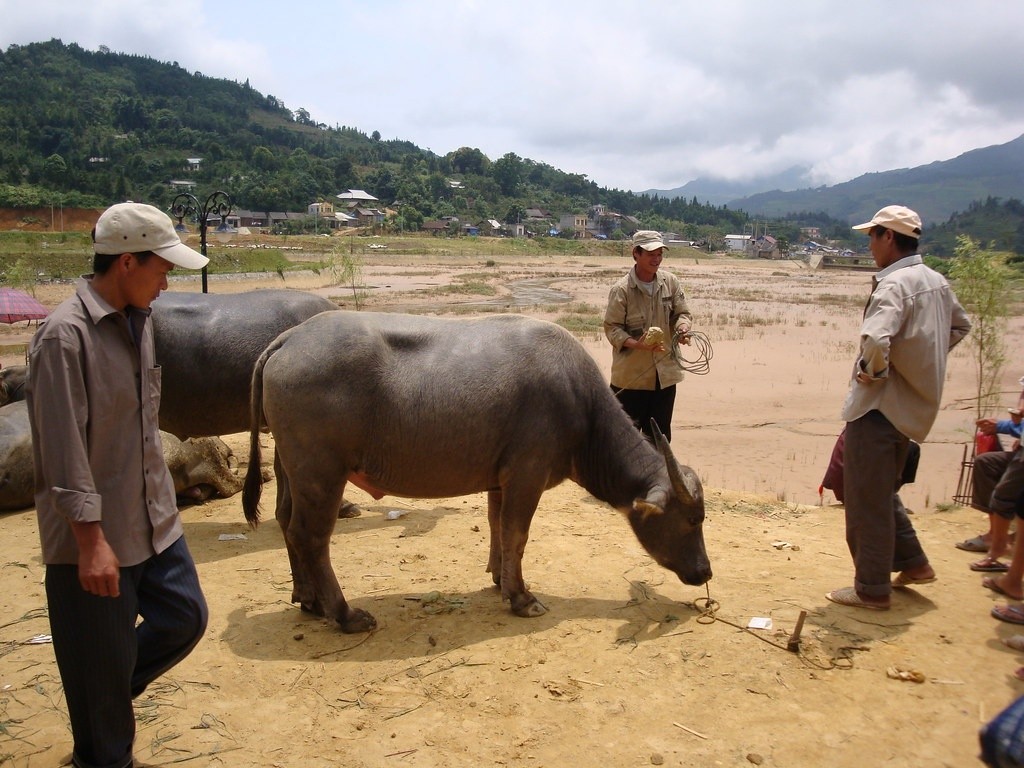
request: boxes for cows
[0,291,362,520]
[242,310,713,634]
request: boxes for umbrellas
[0,286,49,324]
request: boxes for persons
[826,204,972,610]
[603,230,694,446]
[1001,635,1024,680]
[970,372,1024,573]
[982,484,1024,625]
[23,201,208,767]
[955,412,1024,552]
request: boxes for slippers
[892,572,937,587]
[972,559,1008,571]
[983,579,1024,599]
[956,535,989,552]
[1002,634,1024,652]
[826,588,889,611]
[992,606,1024,624]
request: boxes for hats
[632,231,669,251]
[852,205,921,239]
[92,201,209,269]
